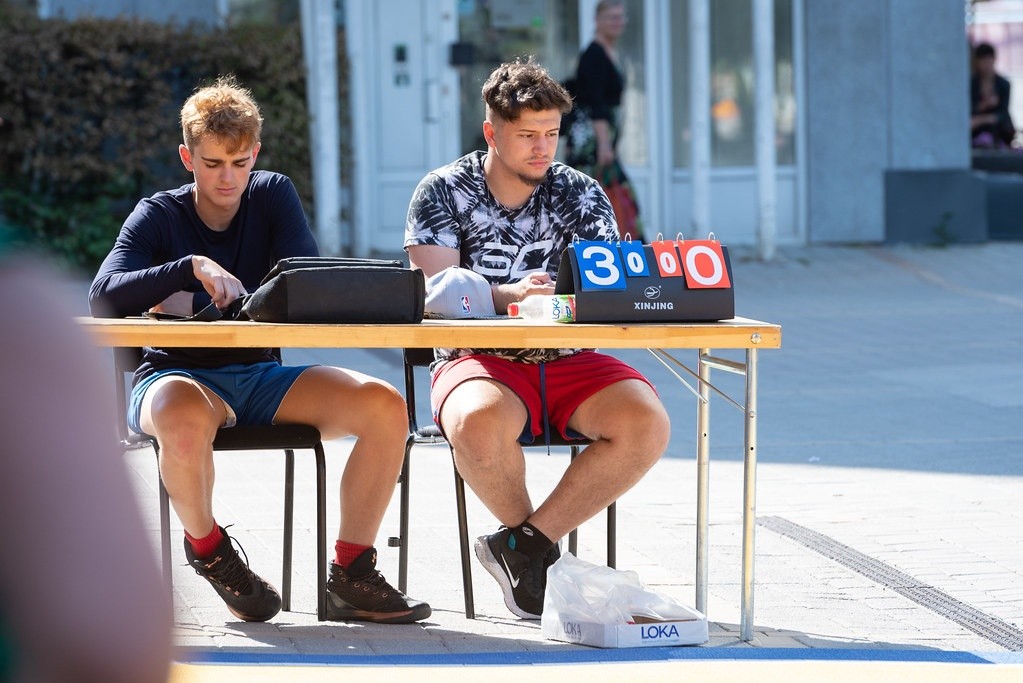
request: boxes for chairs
[114,348,328,621]
[401,348,616,621]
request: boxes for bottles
[508,293,577,322]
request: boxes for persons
[88,87,431,625]
[970,43,1015,148]
[563,0,648,245]
[403,62,670,620]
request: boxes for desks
[74,315,783,640]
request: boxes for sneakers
[327,548,432,624]
[474,525,562,619]
[180,524,281,622]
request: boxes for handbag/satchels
[144,257,425,323]
[591,159,640,244]
[559,79,581,137]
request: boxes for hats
[424,265,524,319]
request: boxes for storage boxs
[541,614,710,646]
